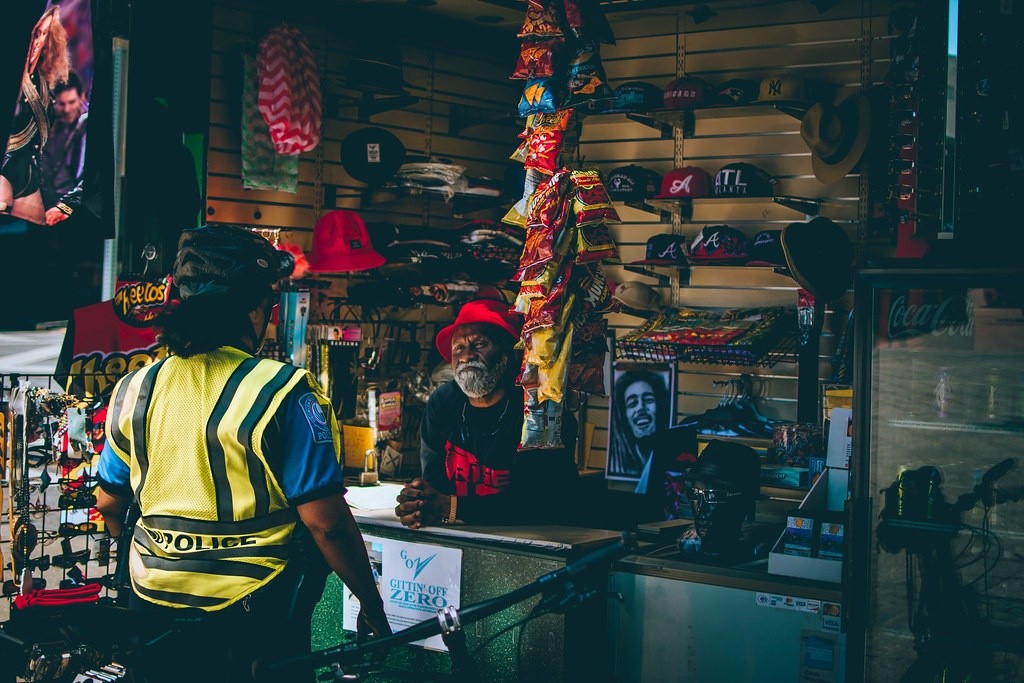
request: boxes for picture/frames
[605,360,678,481]
[600,328,618,398]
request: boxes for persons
[685,440,761,553]
[395,300,581,531]
[1,5,91,224]
[612,371,669,475]
[95,225,392,683]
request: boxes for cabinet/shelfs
[609,288,854,503]
[588,100,811,285]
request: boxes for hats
[611,281,661,318]
[340,126,407,183]
[714,161,769,198]
[607,164,661,201]
[800,93,873,185]
[767,422,825,469]
[664,439,770,500]
[631,224,786,266]
[435,299,522,364]
[172,223,295,300]
[297,209,387,273]
[780,216,849,300]
[653,166,714,199]
[601,74,802,115]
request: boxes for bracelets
[448,494,457,525]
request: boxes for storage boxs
[827,468,848,511]
[824,407,852,470]
[342,424,391,469]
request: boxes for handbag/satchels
[387,321,421,365]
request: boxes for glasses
[272,289,280,305]
[886,0,1022,229]
[1,383,120,594]
